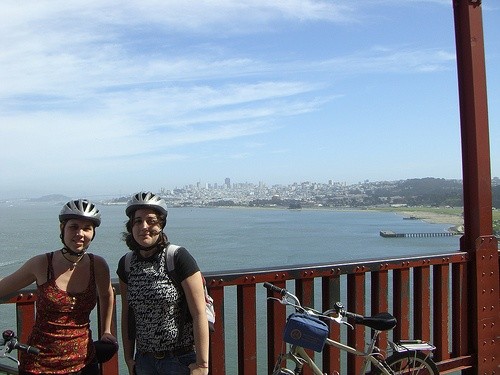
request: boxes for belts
[142,345,195,359]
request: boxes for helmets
[59,199,101,227]
[125,191,168,217]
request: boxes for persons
[0,199,117,375]
[116,191,209,375]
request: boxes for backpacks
[125,244,216,333]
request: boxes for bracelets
[195,363,207,368]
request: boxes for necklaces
[62,252,84,265]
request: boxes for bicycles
[262,282,440,375]
[0,330,117,375]
